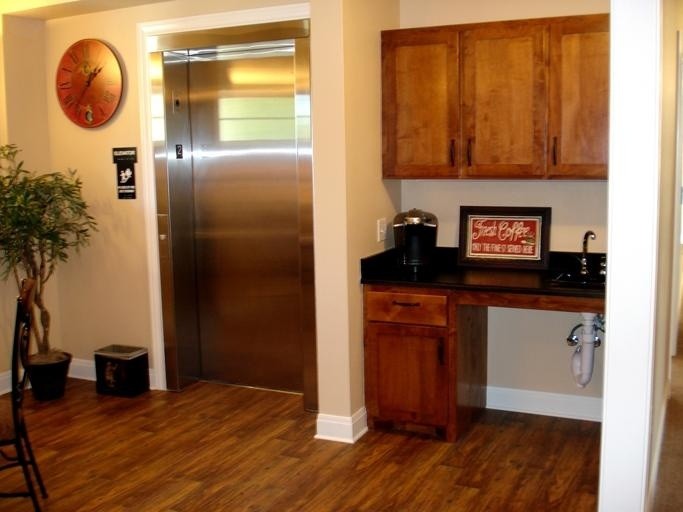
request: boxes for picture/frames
[457,205,552,269]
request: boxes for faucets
[579,231,596,271]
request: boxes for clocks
[55,38,123,128]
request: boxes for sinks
[551,270,607,292]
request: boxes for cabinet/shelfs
[363,291,488,443]
[381,18,551,179]
[548,12,610,179]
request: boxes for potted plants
[0,142,100,402]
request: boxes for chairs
[0,278,50,512]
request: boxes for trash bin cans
[93,344,150,396]
